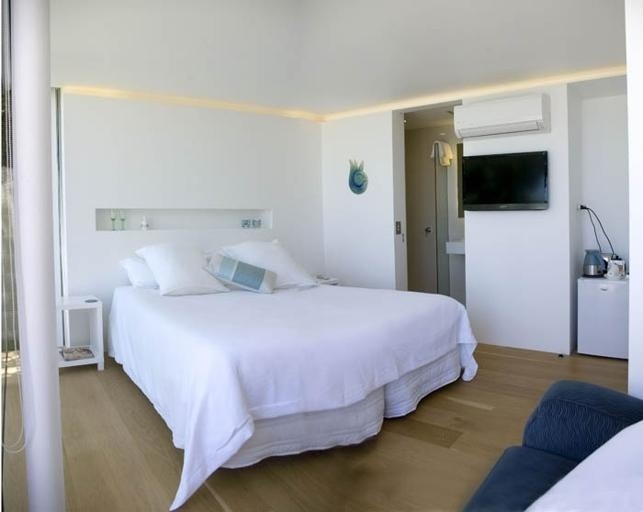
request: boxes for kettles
[582,248,605,278]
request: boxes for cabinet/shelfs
[576,275,627,362]
[54,293,105,372]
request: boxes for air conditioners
[450,92,553,142]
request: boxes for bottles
[141,215,149,231]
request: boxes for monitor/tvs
[461,150,550,210]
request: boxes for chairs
[462,379,643,510]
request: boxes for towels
[430,139,454,169]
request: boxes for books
[59,348,94,362]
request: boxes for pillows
[524,417,643,509]
[121,235,316,298]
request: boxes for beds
[113,280,472,472]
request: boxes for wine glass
[117,209,126,231]
[109,208,117,231]
